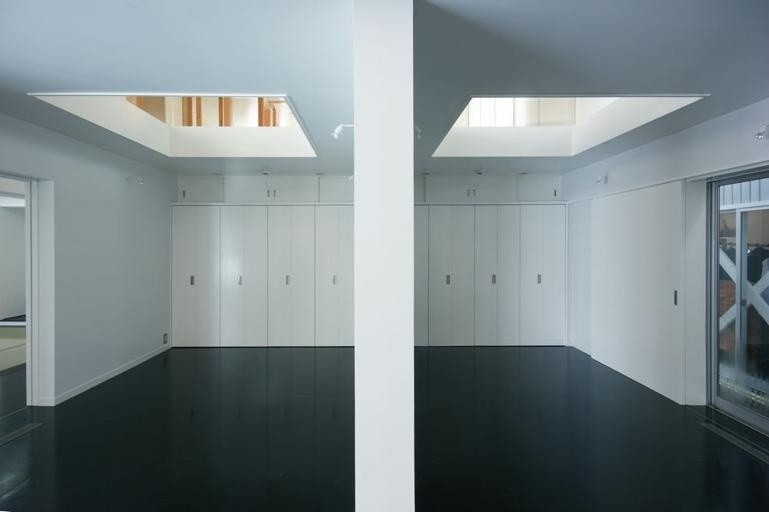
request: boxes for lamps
[330,123,354,140]
